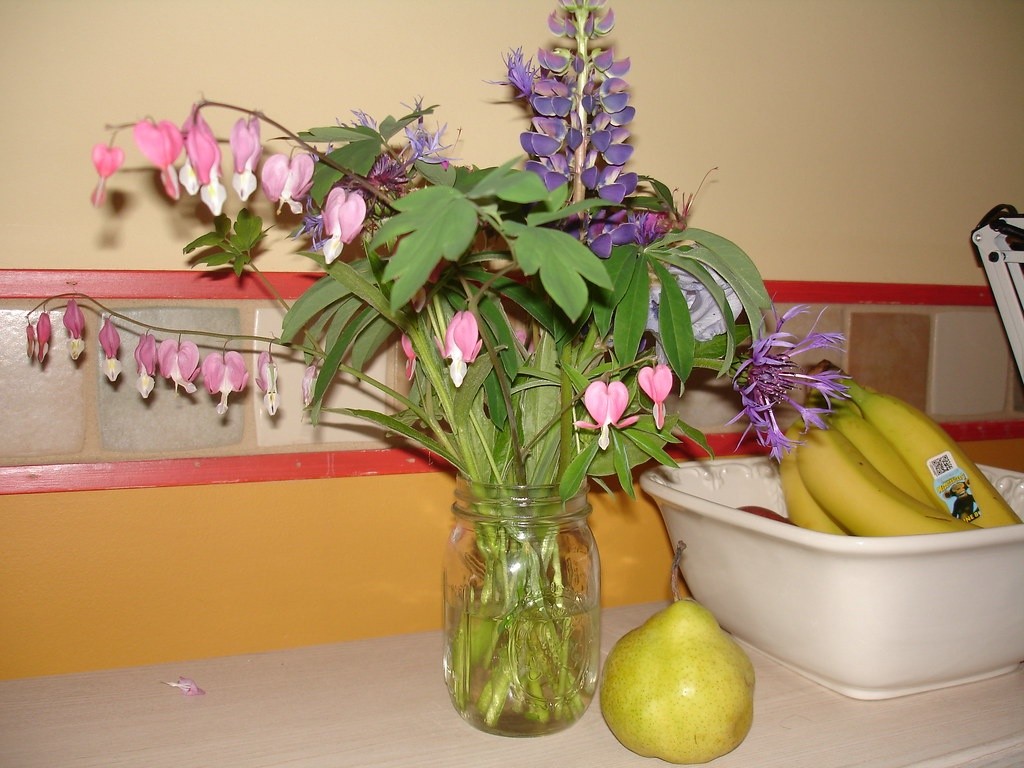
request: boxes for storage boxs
[639,454,1024,701]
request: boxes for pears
[599,540,754,764]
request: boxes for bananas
[780,360,1024,537]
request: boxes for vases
[441,473,602,738]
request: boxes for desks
[0,596,1024,768]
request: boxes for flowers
[24,0,867,731]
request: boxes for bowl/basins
[638,454,1024,701]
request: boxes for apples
[739,505,798,525]
